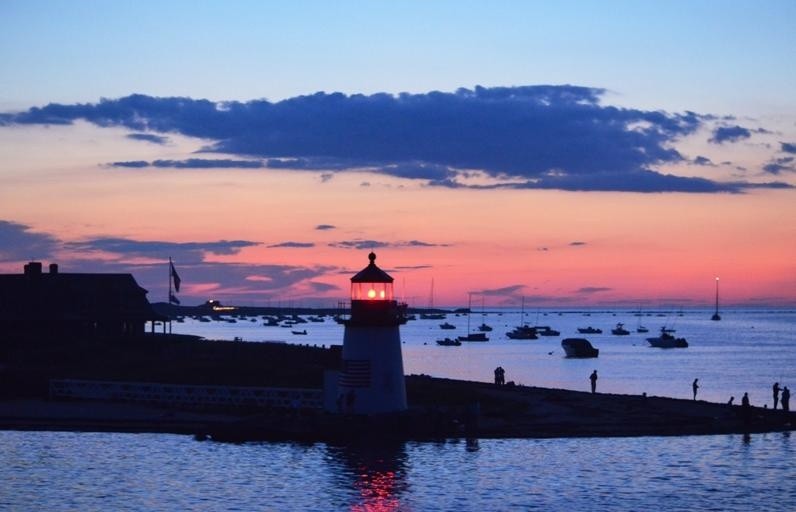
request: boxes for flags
[171,265,181,292]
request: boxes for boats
[175,301,348,335]
[560,338,600,358]
[505,295,688,340]
[397,277,491,346]
[646,335,687,350]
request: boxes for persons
[742,392,750,407]
[499,367,505,385]
[589,370,597,394]
[728,397,734,406]
[494,367,500,384]
[693,378,699,401]
[781,386,790,410]
[773,382,782,409]
[764,404,767,409]
